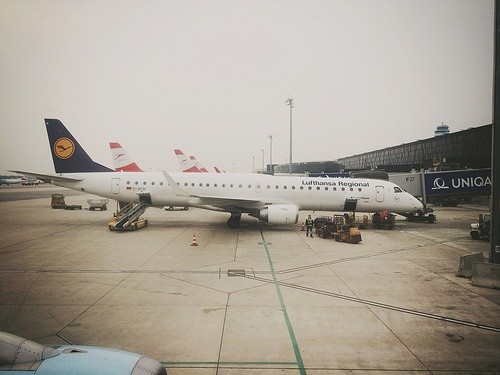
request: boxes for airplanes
[0,119,423,224]
[0,330,167,374]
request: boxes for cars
[165,206,188,211]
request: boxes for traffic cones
[190,234,198,246]
[301,222,305,232]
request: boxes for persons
[306,215,314,238]
